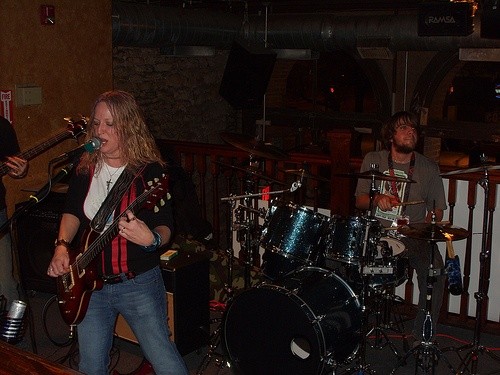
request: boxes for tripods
[345,167,500,375]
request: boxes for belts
[100,260,160,286]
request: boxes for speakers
[15,192,84,296]
[419,2,473,37]
[113,250,211,356]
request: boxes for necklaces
[104,164,119,190]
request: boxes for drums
[222,198,409,375]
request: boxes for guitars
[56,172,170,328]
[0,112,90,178]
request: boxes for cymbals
[224,136,292,161]
[397,222,470,242]
[276,164,328,181]
[336,170,417,183]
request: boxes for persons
[355,111,446,366]
[0,117,29,312]
[47,91,190,375]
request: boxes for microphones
[50,137,102,166]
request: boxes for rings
[130,217,136,221]
[121,227,125,233]
[50,269,54,271]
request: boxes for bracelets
[144,231,161,252]
[55,239,69,248]
